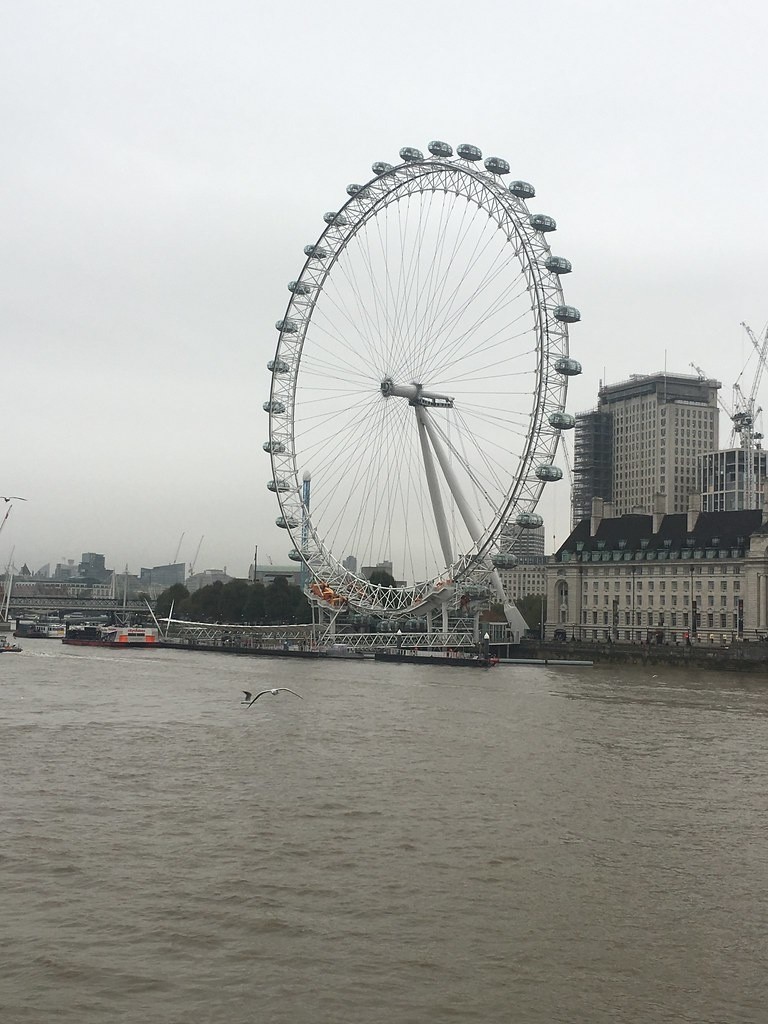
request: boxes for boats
[14,625,46,639]
[0,634,23,653]
[62,624,161,647]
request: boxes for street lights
[122,564,130,621]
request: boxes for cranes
[690,320,768,510]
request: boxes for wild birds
[238,688,305,712]
[0,494,30,504]
